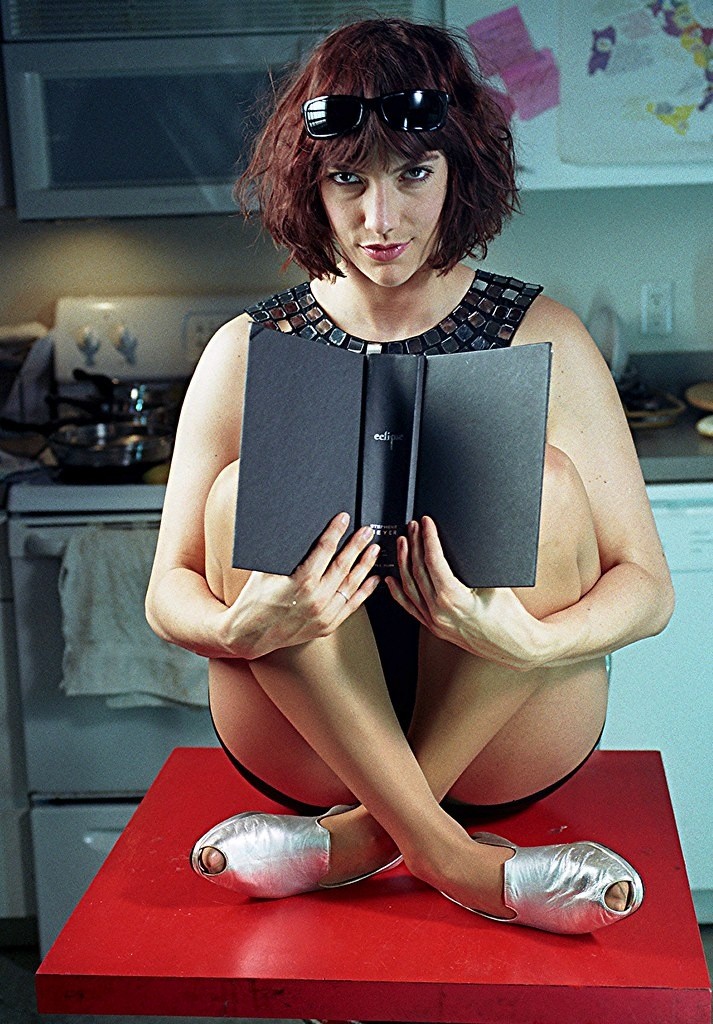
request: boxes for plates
[683,381,713,412]
[696,415,713,437]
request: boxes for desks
[37,747,713,1024]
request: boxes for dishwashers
[5,510,238,963]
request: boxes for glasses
[300,88,456,140]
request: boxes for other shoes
[189,805,403,900]
[439,831,643,936]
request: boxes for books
[236,322,550,589]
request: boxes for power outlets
[640,281,673,335]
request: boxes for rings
[339,592,350,601]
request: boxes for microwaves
[1,0,444,222]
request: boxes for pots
[71,366,175,414]
[44,420,175,472]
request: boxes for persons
[142,18,674,936]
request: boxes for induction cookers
[5,292,289,511]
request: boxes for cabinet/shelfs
[444,0,713,191]
[7,506,713,965]
